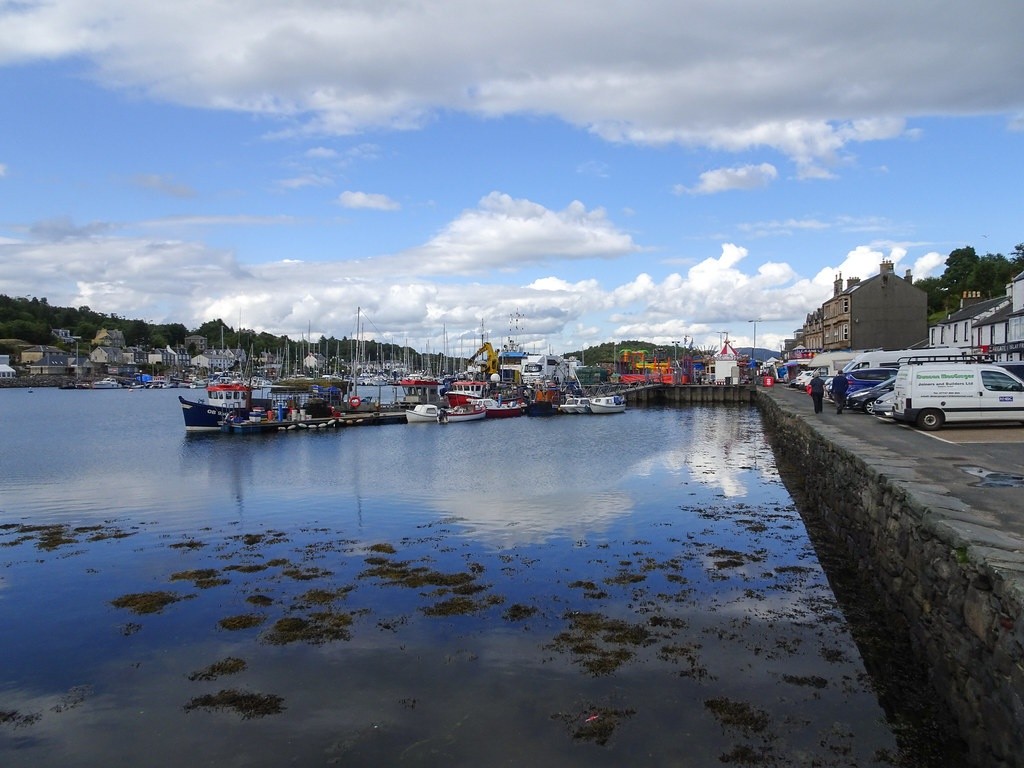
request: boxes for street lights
[672,341,680,371]
[717,331,728,349]
[749,320,762,352]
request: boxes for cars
[846,376,895,414]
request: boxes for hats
[814,372,820,378]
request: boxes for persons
[832,369,849,415]
[809,371,825,414]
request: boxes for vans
[891,354,1024,431]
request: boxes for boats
[179,306,626,431]
[28,387,33,393]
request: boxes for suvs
[789,371,814,387]
[843,369,898,404]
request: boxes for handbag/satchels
[807,384,813,396]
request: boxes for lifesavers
[349,396,361,408]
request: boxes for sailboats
[92,299,440,389]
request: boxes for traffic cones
[799,348,986,401]
